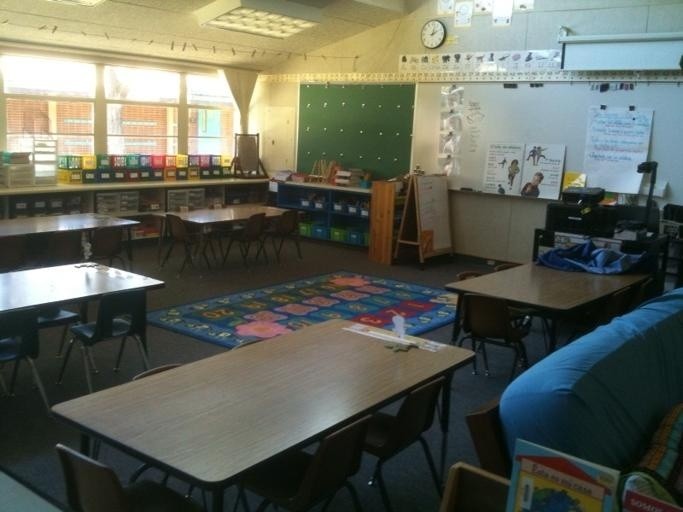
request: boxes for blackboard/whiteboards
[409,80,683,207]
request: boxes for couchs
[464,287,682,511]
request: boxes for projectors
[562,187,605,205]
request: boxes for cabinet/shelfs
[0,176,270,246]
[269,180,414,251]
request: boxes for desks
[165,203,305,273]
[52,317,477,512]
[445,258,653,383]
[1,261,166,398]
[0,212,141,273]
[533,227,669,299]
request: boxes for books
[622,488,682,511]
[506,438,620,512]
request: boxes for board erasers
[460,187,472,191]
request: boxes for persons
[498,145,548,198]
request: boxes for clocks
[420,20,445,48]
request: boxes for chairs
[133,363,181,379]
[91,227,128,271]
[219,209,304,272]
[493,264,559,350]
[363,374,446,512]
[456,271,523,320]
[462,293,532,387]
[55,443,207,511]
[160,214,213,277]
[243,414,374,512]
[0,288,145,416]
[564,276,657,346]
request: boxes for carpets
[147,266,459,349]
